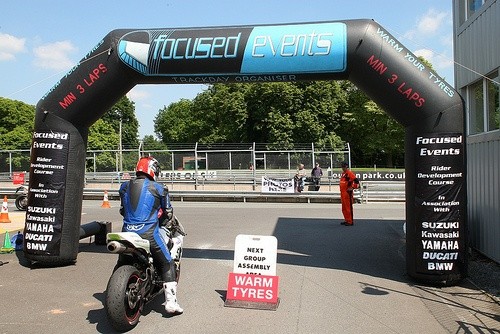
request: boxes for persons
[311,163,323,191]
[118,157,183,316]
[295,163,306,192]
[339,161,360,226]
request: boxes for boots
[161,282,184,317]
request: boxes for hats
[340,161,348,167]
[315,162,319,164]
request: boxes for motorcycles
[106,209,187,332]
[15,181,29,210]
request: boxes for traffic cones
[0,195,11,223]
[101,191,112,210]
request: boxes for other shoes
[341,221,354,226]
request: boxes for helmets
[135,157,161,181]
